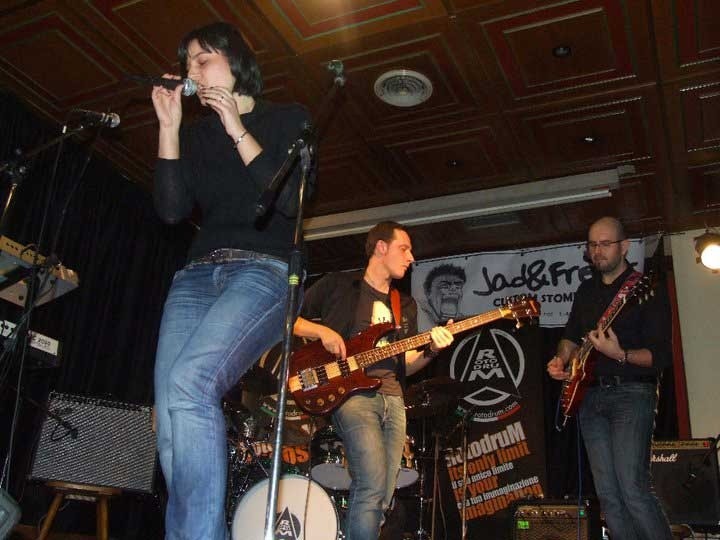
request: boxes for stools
[36,481,122,540]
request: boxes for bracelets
[619,350,629,364]
[424,345,437,358]
[233,131,248,149]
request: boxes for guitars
[559,270,656,419]
[286,294,542,418]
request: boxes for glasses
[586,240,624,248]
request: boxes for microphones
[126,72,198,96]
[74,108,120,128]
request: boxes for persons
[144,20,302,540]
[547,217,672,540]
[288,224,454,540]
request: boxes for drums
[311,427,353,514]
[230,472,340,540]
[394,434,419,500]
